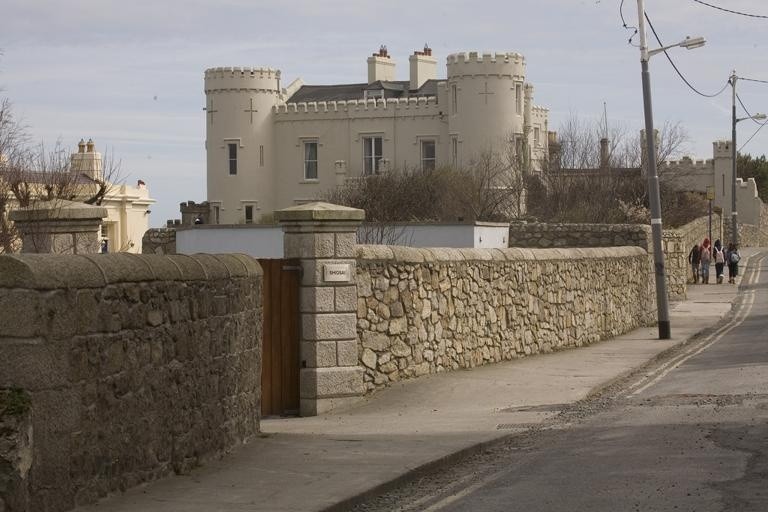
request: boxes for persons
[195,212,204,223]
[688,238,741,284]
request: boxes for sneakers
[701,275,734,284]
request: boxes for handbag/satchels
[729,253,740,264]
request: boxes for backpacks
[701,245,710,263]
[714,246,725,264]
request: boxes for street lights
[727,111,767,282]
[632,32,703,338]
